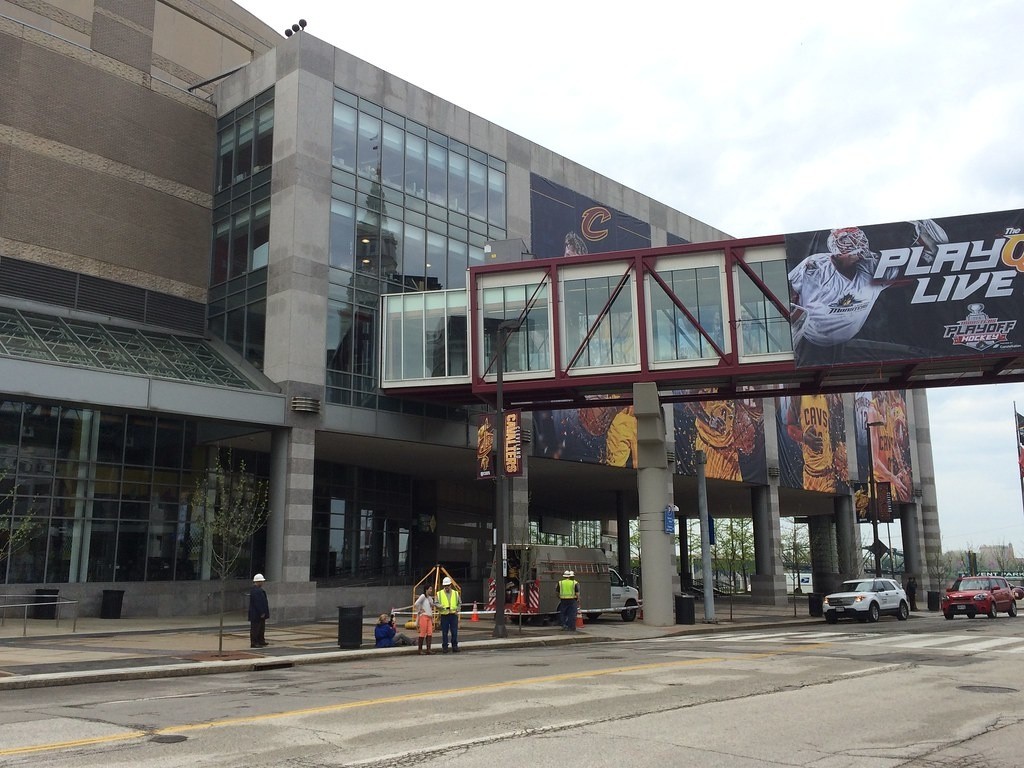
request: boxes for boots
[426,636,436,654]
[418,637,426,654]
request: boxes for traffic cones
[514,584,525,605]
[575,604,585,628]
[467,601,480,621]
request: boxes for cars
[1009,583,1024,600]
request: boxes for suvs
[823,577,911,622]
[941,576,1017,620]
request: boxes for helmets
[252,574,265,581]
[441,577,452,585]
[563,570,570,577]
[570,571,576,576]
[827,228,870,263]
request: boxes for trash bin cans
[808,592,823,617]
[336,604,364,649]
[35,589,60,620]
[676,595,695,624]
[101,589,126,619]
[927,591,940,611]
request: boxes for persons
[563,232,588,256]
[375,614,416,648]
[248,574,270,649]
[685,387,742,481]
[555,570,581,631]
[907,577,920,611]
[415,583,436,655]
[867,391,908,500]
[788,215,949,365]
[595,393,638,468]
[434,577,462,653]
[787,395,841,492]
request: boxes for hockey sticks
[790,231,822,338]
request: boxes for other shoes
[250,644,263,648]
[410,640,416,645]
[259,641,269,645]
[453,647,460,652]
[402,641,407,645]
[561,624,566,631]
[443,647,448,653]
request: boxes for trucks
[484,543,639,626]
